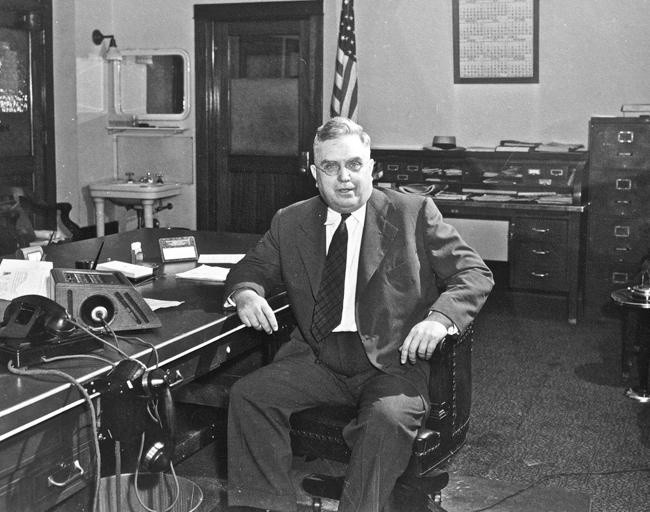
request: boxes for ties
[311,213,352,342]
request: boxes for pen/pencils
[91,242,104,270]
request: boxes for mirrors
[112,48,192,122]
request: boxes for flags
[327,2,362,131]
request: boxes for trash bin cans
[99,472,204,512]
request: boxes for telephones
[105,360,176,473]
[0,295,104,368]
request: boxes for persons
[221,115,497,512]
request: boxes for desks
[2,226,299,482]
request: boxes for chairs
[267,319,473,512]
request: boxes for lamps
[92,29,123,61]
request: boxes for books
[620,102,650,112]
[623,112,649,117]
[495,145,530,153]
[500,138,541,148]
[94,258,154,279]
[396,180,575,209]
[533,142,585,153]
[175,264,232,283]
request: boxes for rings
[254,325,262,331]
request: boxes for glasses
[315,158,370,176]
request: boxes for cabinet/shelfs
[0,407,100,512]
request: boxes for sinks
[88,177,181,200]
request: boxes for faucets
[138,172,154,184]
